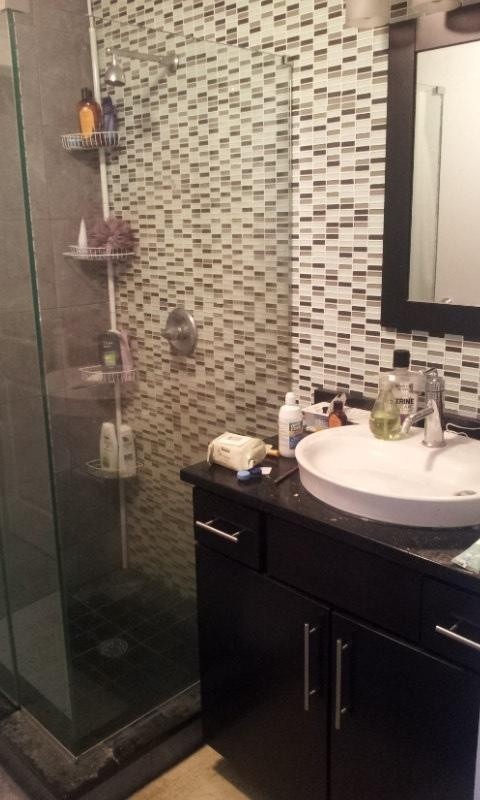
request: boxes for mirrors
[373,0,479,342]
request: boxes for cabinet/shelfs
[185,476,480,800]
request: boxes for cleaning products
[76,86,118,146]
[278,391,305,461]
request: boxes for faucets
[400,367,447,447]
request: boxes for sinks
[292,425,478,530]
